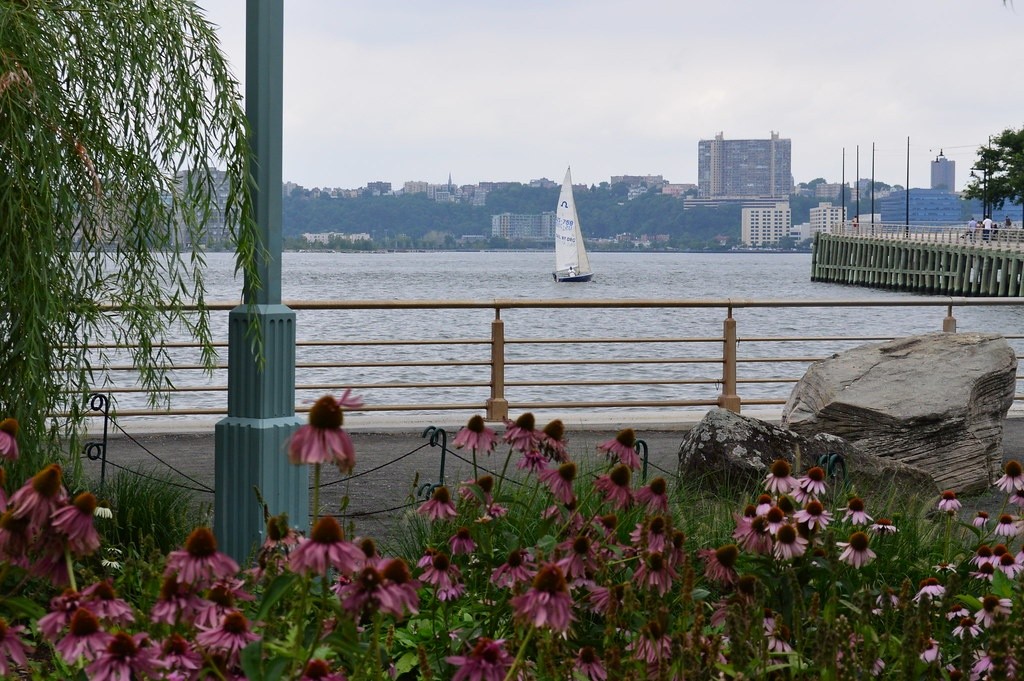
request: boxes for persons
[852,216,857,233]
[568,267,576,277]
[552,271,558,282]
[1005,215,1011,228]
[969,216,998,244]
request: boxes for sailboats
[552,165,594,283]
